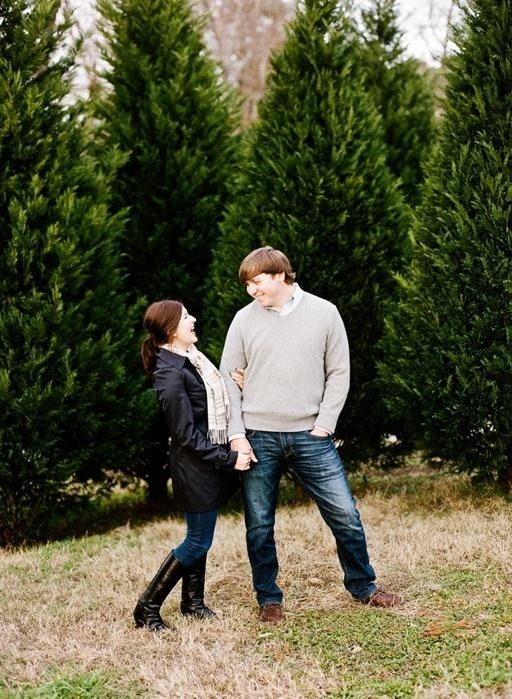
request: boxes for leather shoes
[261,604,281,621]
[366,590,401,607]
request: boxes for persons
[219,246,404,626]
[134,300,254,631]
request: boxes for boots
[181,552,216,618]
[133,549,182,629]
